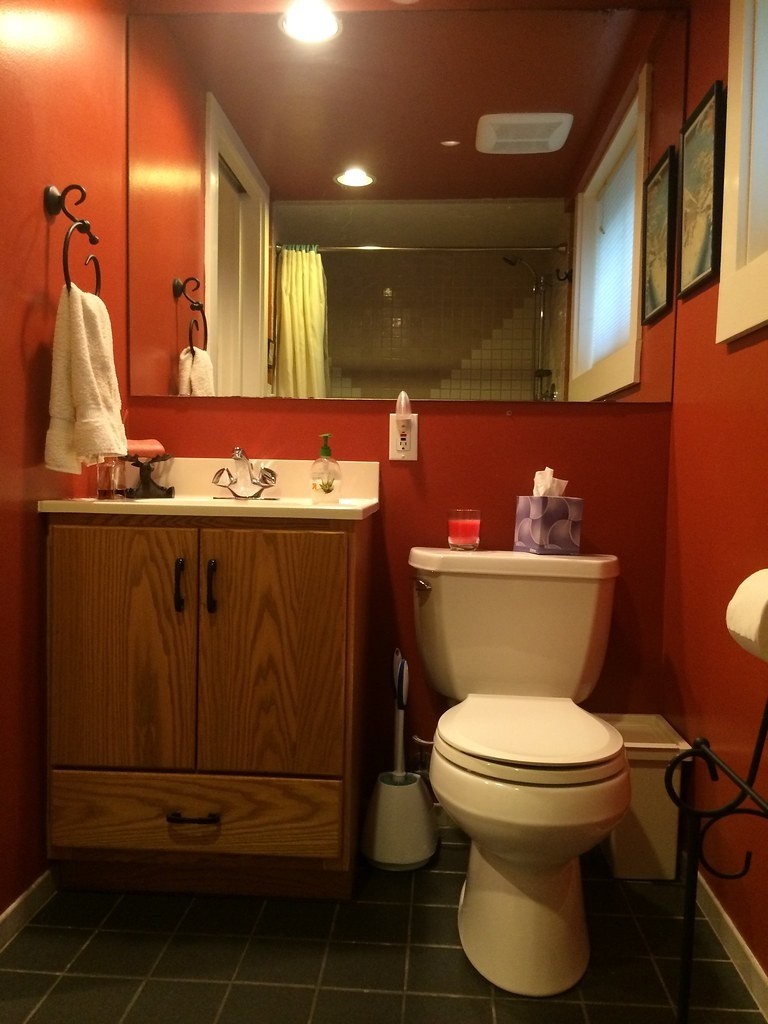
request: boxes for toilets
[404,544,634,1001]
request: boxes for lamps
[395,390,411,433]
[279,0,340,45]
[334,157,377,187]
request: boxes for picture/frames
[640,145,678,326]
[675,77,727,297]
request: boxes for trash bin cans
[592,712,695,879]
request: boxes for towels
[43,282,129,476]
[177,347,217,397]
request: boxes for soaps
[119,438,167,459]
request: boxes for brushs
[380,659,417,785]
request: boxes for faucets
[230,446,257,499]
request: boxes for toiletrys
[308,433,342,506]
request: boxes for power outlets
[388,414,419,462]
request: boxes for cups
[96,455,127,501]
[447,508,481,551]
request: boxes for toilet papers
[725,565,767,661]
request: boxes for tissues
[512,466,584,558]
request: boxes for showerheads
[501,254,542,287]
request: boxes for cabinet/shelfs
[37,496,380,873]
